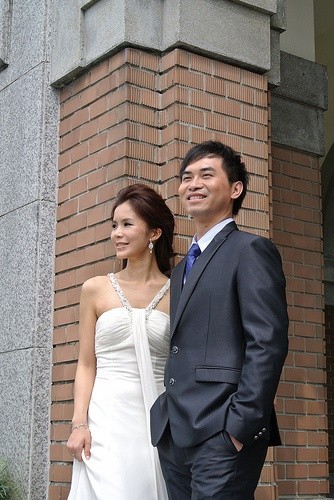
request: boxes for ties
[184,244,201,282]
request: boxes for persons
[149,140,291,500]
[65,182,181,499]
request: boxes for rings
[72,454,76,457]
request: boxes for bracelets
[71,424,89,432]
